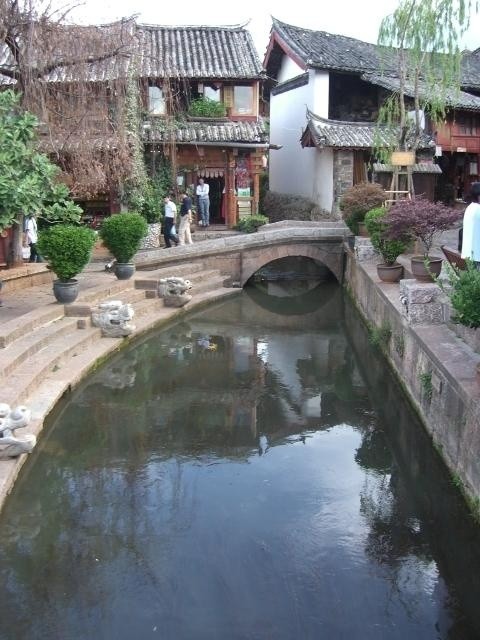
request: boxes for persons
[163,196,179,249]
[461,182,480,263]
[179,190,194,245]
[196,179,211,227]
[25,213,42,263]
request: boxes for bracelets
[188,219,192,221]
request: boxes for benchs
[441,243,472,277]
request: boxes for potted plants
[187,96,229,121]
[339,181,387,238]
[373,196,465,282]
[36,224,98,304]
[99,211,148,280]
[364,205,412,283]
